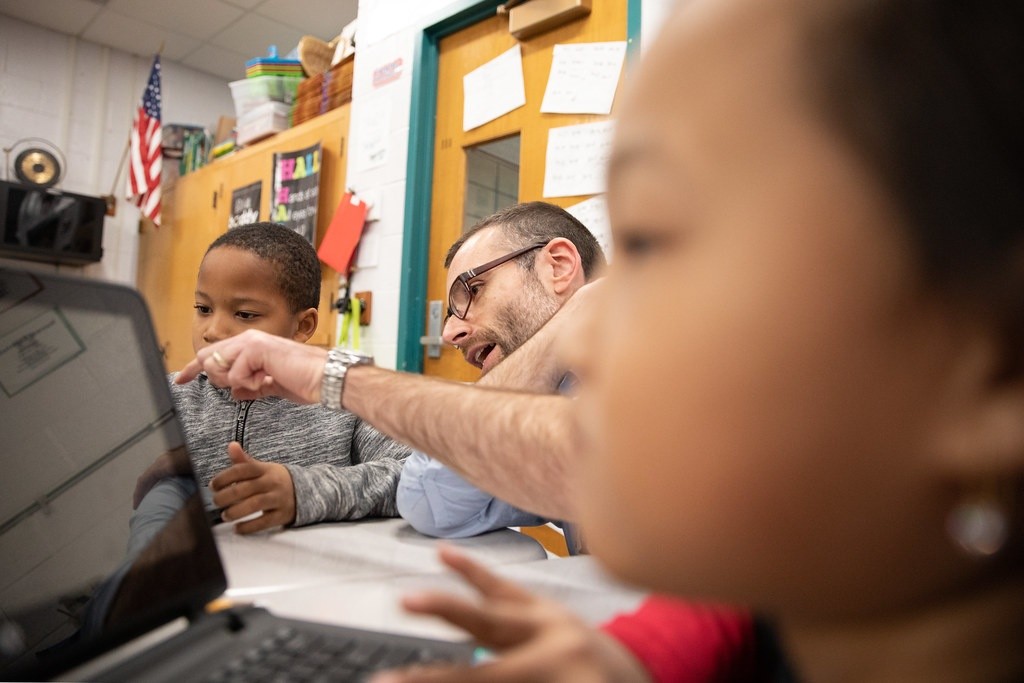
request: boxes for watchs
[321,346,374,413]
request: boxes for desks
[206,504,551,602]
[256,551,651,662]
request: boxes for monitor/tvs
[0,180,105,266]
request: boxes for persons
[369,0,1024,683]
[165,222,413,536]
[174,200,608,557]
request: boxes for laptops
[0,267,479,683]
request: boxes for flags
[125,55,163,229]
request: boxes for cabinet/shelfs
[236,102,350,352]
[134,148,235,375]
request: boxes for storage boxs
[227,43,355,147]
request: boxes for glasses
[444,242,547,324]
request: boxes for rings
[212,352,230,370]
